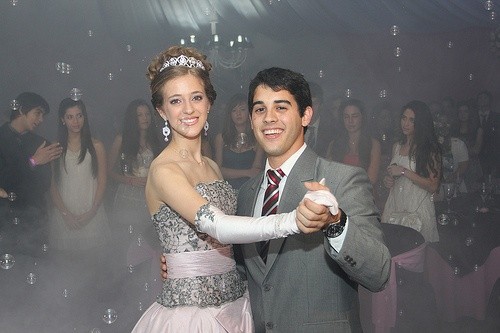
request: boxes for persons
[0,92,64,248]
[108,100,158,224]
[50,98,111,252]
[201,93,264,195]
[130,46,340,333]
[380,101,440,243]
[161,67,391,333]
[305,81,500,196]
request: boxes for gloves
[194,177,339,243]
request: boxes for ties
[260,168,285,264]
[481,113,487,129]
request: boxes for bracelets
[29,158,36,165]
[401,168,406,176]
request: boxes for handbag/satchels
[388,208,422,232]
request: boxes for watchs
[323,208,347,238]
